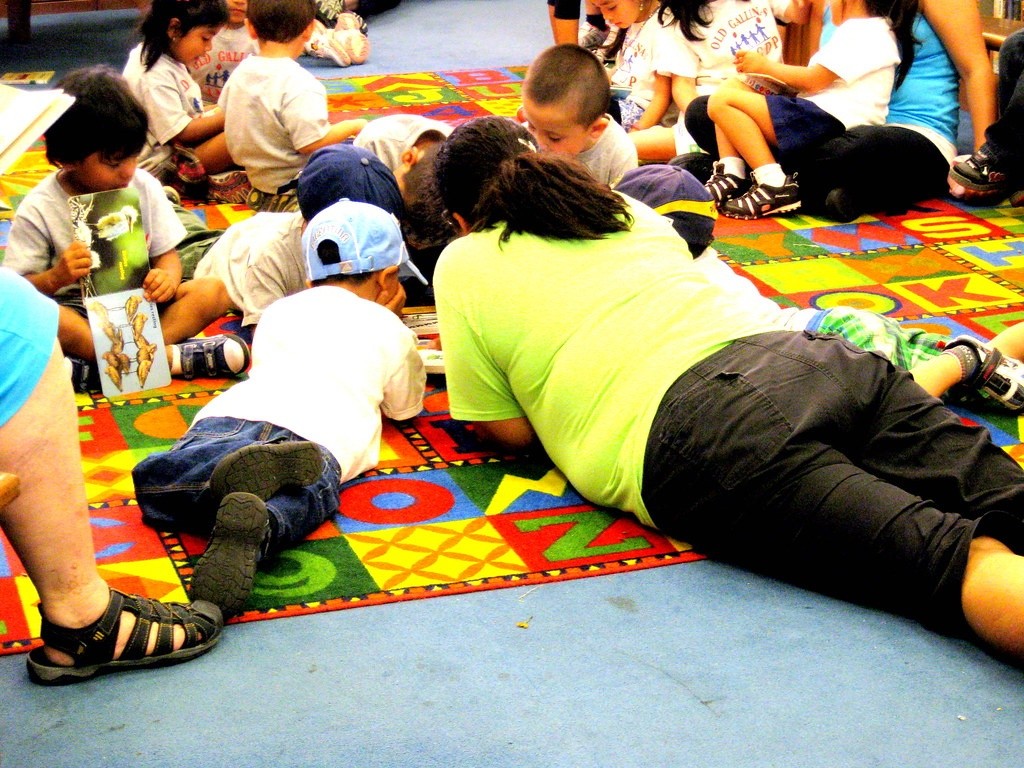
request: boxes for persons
[614,165,1024,410]
[431,115,1024,656]
[547,0,1024,224]
[521,45,637,189]
[0,0,462,686]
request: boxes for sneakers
[175,334,250,379]
[189,492,267,615]
[702,161,750,207]
[949,143,1015,189]
[211,442,323,501]
[333,13,369,65]
[303,19,351,67]
[64,357,101,393]
[944,335,1024,409]
[723,174,801,219]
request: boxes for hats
[301,198,428,285]
[277,144,404,223]
[613,164,719,258]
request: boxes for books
[66,188,171,399]
[399,313,445,373]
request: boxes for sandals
[26,588,221,686]
